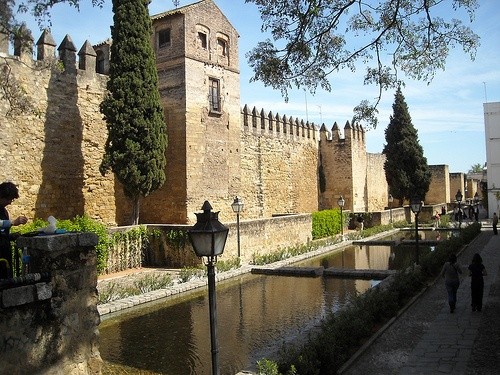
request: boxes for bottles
[22,247,32,277]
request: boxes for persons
[455,205,479,222]
[357,213,364,230]
[436,211,440,227]
[442,206,446,215]
[493,212,498,235]
[0,182,28,279]
[441,254,462,313]
[468,253,487,313]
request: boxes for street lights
[186,199,230,375]
[231,196,244,258]
[336,195,344,237]
[474,191,481,220]
[455,188,463,232]
[408,199,423,265]
